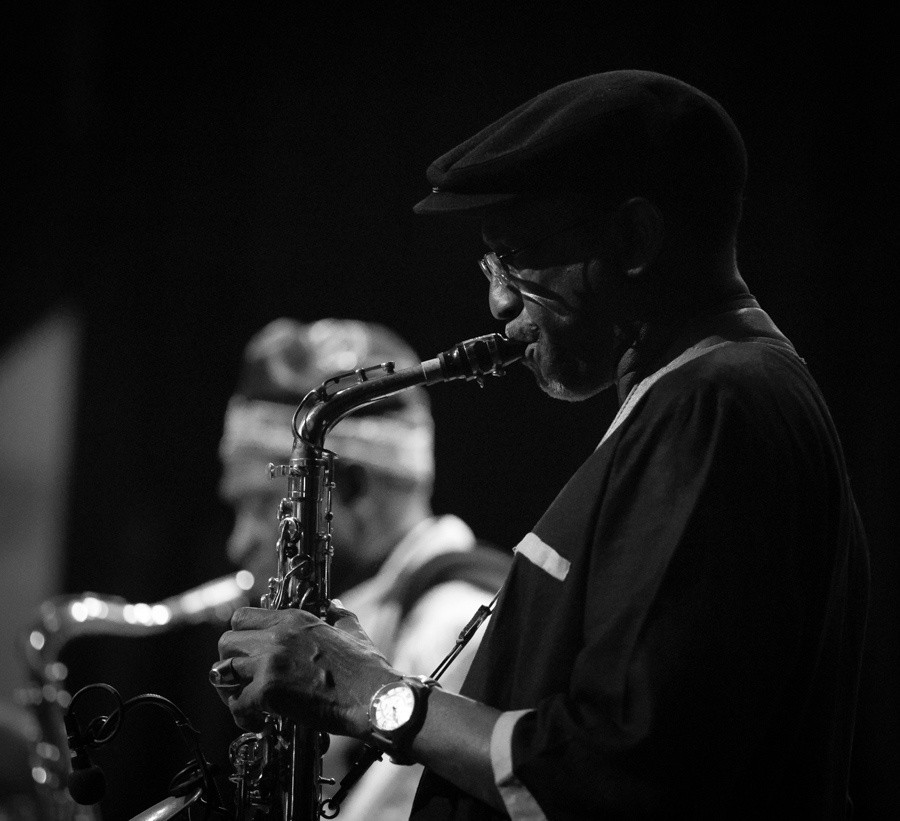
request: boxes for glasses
[473,203,630,289]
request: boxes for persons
[41,317,516,820]
[213,69,869,820]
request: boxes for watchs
[368,676,441,766]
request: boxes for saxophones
[129,335,528,821]
[1,572,252,821]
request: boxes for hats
[413,65,749,214]
[217,321,437,483]
